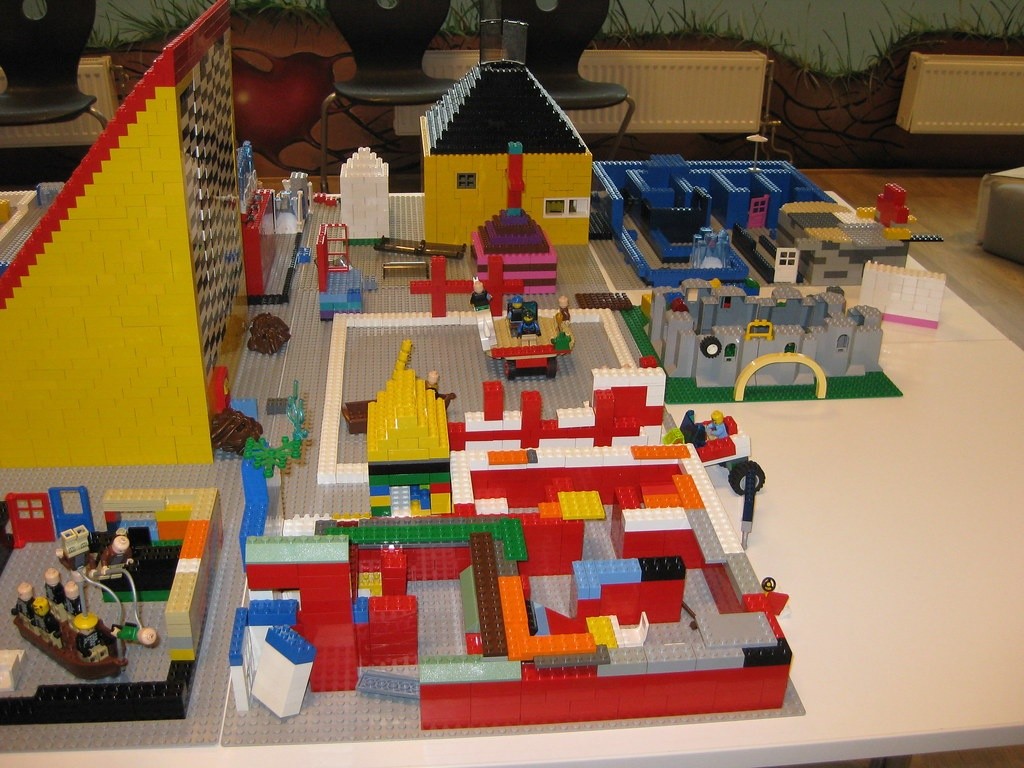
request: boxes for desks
[0,192,1022,766]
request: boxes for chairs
[0,0,107,129]
[320,0,459,193]
[499,0,635,161]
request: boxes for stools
[973,166,1024,265]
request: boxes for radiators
[894,52,1024,135]
[393,46,767,133]
[0,54,119,148]
